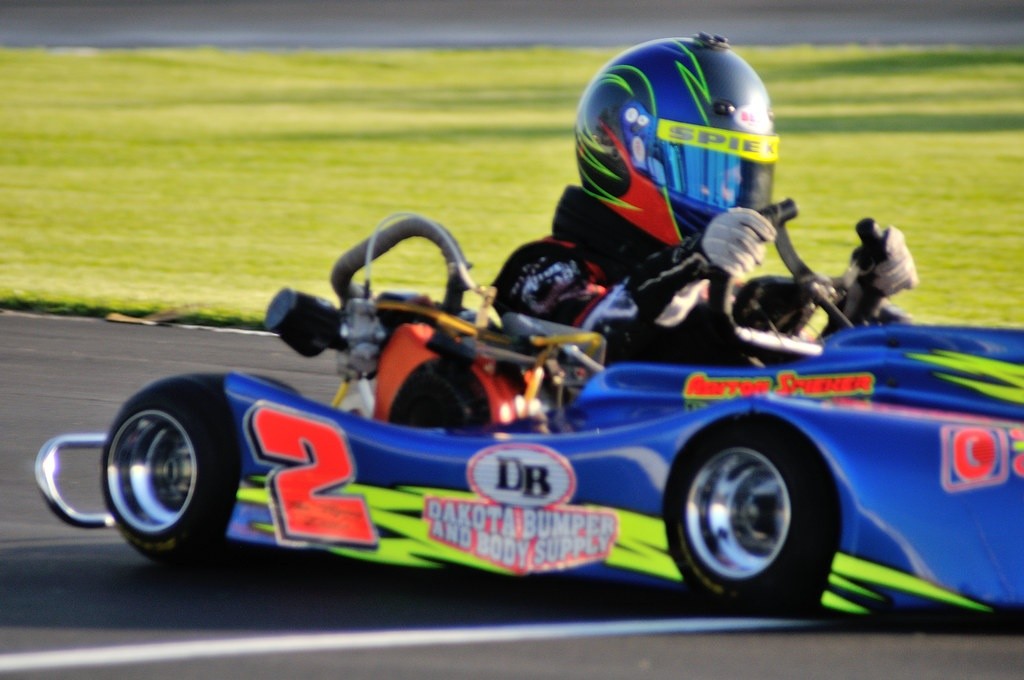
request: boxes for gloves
[851,226,918,299]
[702,207,778,277]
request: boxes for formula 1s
[34,33,1024,635]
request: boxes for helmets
[575,31,779,249]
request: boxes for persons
[489,34,921,429]
